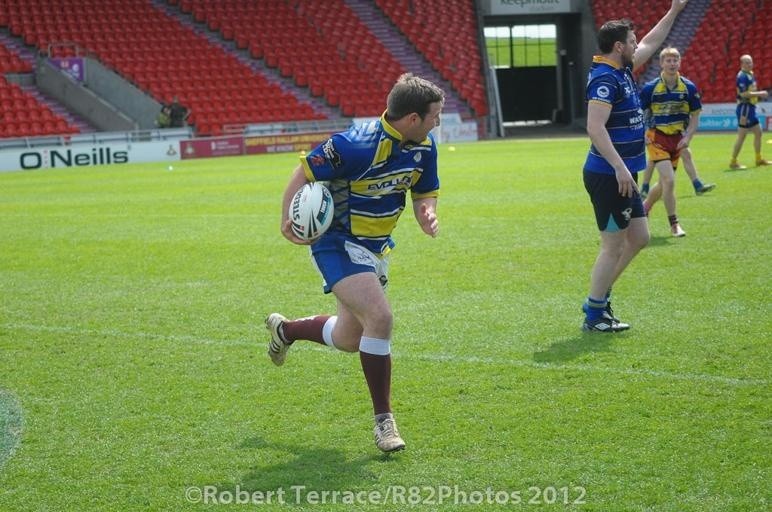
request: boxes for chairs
[590,0,772,103]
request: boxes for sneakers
[670,225,685,238]
[695,182,716,194]
[641,189,649,197]
[582,298,630,333]
[756,158,772,166]
[729,163,748,170]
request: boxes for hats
[373,417,406,454]
[265,312,294,367]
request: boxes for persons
[580,0,688,333]
[158,104,171,128]
[164,95,191,128]
[640,113,716,196]
[638,46,702,238]
[729,54,772,170]
[264,71,446,455]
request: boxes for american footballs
[290,181,334,240]
